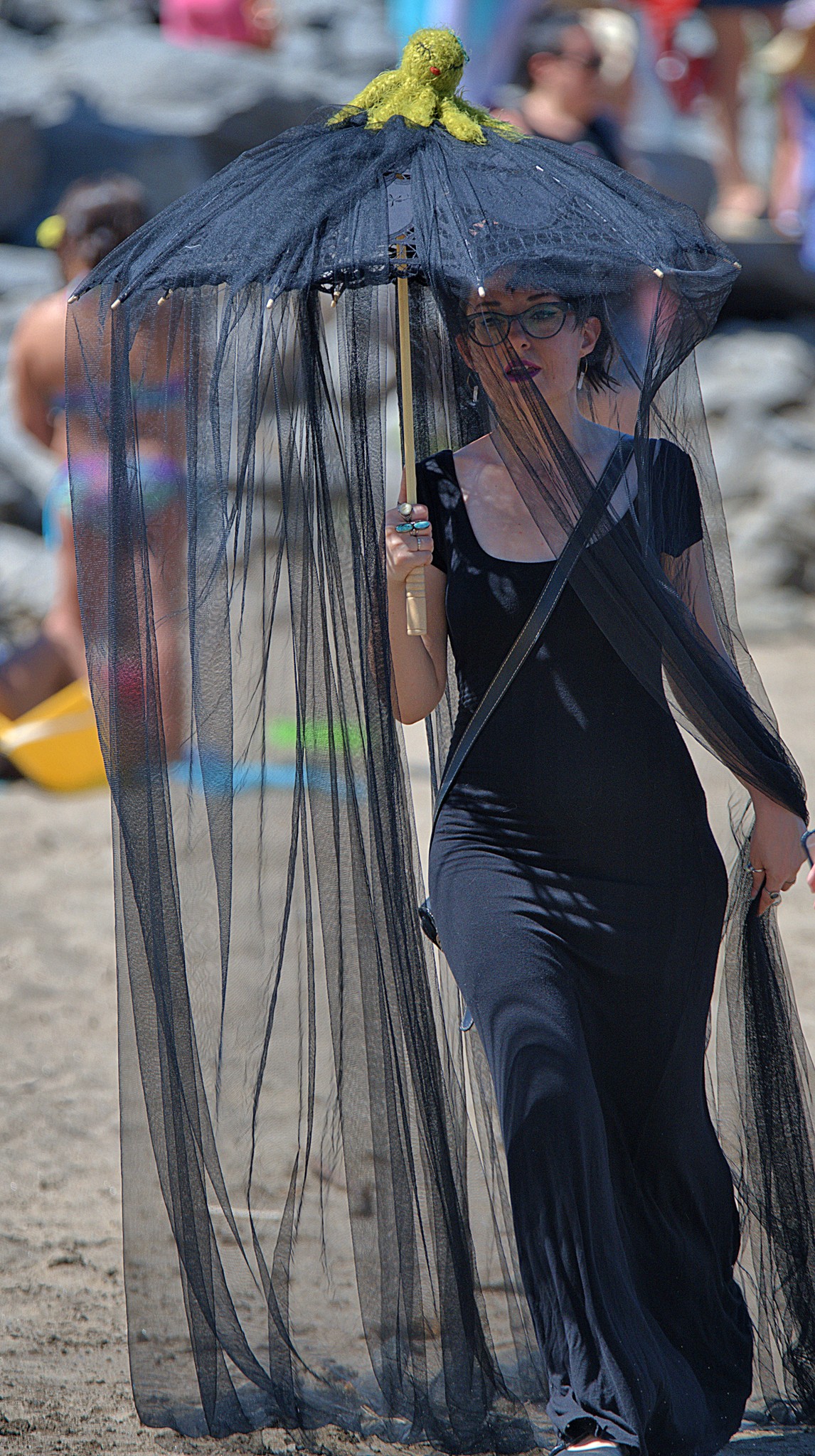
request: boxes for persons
[379,236,815,1456]
[0,0,815,768]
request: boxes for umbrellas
[73,32,737,643]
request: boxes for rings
[398,503,413,523]
[764,885,779,899]
[785,879,797,885]
[395,519,430,536]
[414,536,421,551]
[752,868,764,872]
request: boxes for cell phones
[800,829,815,867]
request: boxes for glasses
[463,300,576,347]
[557,51,605,73]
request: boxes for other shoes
[550,1427,626,1455]
